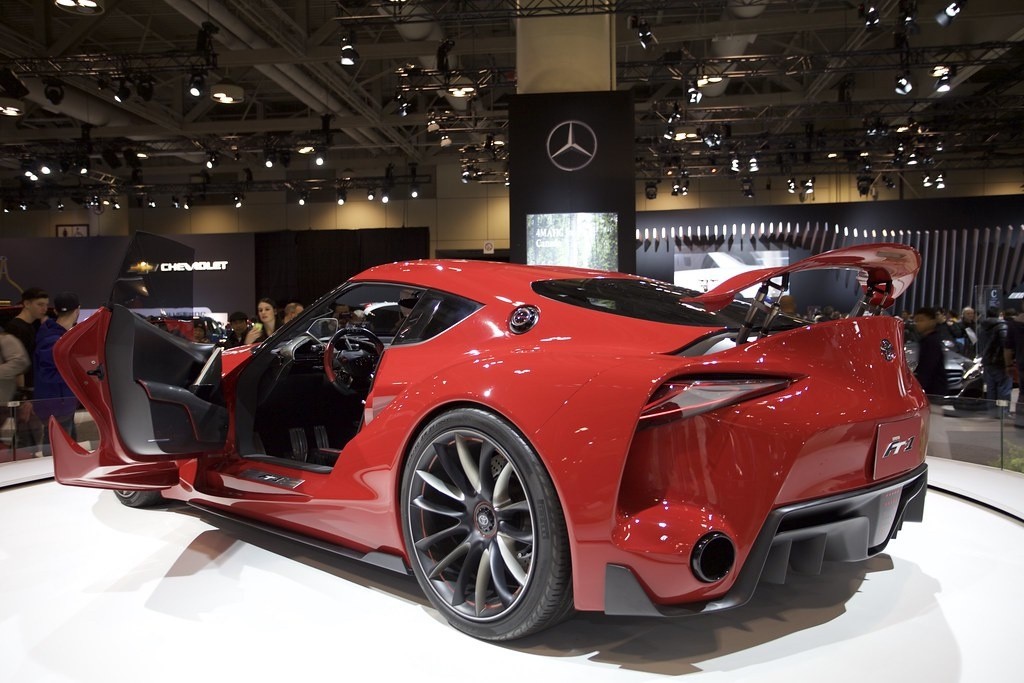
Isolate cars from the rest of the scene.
[0,300,255,345]
[901,329,986,399]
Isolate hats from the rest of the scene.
[10,287,48,305]
[53,292,80,313]
[229,312,249,322]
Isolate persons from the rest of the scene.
[778,294,1024,419]
[0,287,416,458]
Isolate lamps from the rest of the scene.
[637,0,968,200]
[0,0,510,214]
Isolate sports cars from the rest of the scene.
[47,241,933,646]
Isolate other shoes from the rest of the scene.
[1008,413,1017,419]
[1000,407,1008,414]
